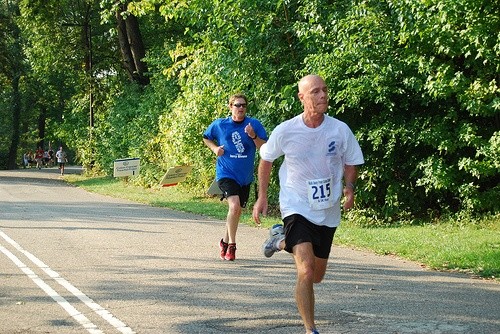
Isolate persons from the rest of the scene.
[202,95,268,261]
[252,74,365,334]
[24,146,67,175]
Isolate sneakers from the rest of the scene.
[219,238,237,261]
[263,223,285,258]
[304,329,322,334]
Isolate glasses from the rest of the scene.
[234,103,248,108]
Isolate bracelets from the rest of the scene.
[347,180,356,191]
[253,134,257,139]
